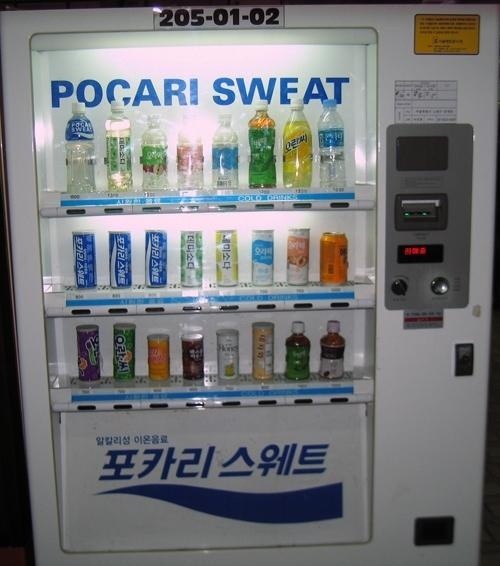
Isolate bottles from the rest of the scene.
[283,97,313,189]
[319,320,345,378]
[248,99,277,188]
[176,128,205,191]
[141,114,168,191]
[105,100,133,193]
[211,112,239,190]
[285,322,311,380]
[65,103,96,192]
[318,99,346,191]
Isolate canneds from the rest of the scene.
[320,233,348,284]
